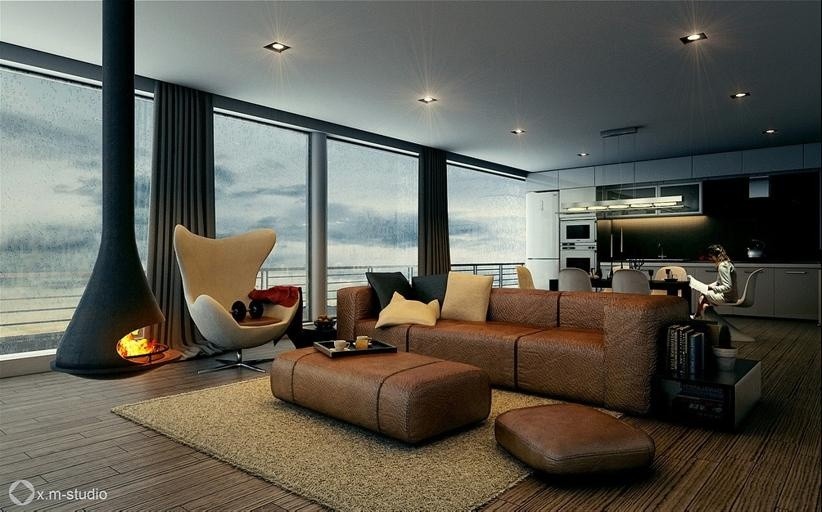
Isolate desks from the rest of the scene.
[549,278,691,315]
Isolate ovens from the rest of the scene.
[560,246,596,276]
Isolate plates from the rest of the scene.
[664,279,678,281]
[352,342,373,346]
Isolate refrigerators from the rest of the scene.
[525,190,559,292]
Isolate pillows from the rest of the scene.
[375,291,440,328]
[411,274,447,309]
[366,272,412,310]
[441,271,494,322]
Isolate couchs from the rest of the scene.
[337,285,689,417]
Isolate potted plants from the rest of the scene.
[712,324,739,371]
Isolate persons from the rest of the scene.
[688,244,739,320]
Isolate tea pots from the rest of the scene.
[746,239,767,258]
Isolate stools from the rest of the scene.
[495,403,655,488]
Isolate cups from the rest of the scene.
[355,339,368,351]
[357,336,373,343]
[333,339,350,351]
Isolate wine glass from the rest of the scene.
[649,269,654,283]
[665,269,672,279]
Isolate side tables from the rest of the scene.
[653,358,762,425]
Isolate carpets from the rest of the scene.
[111,375,624,512]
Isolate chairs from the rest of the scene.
[701,268,764,343]
[651,266,688,297]
[517,266,535,289]
[612,269,650,295]
[558,268,592,291]
[173,224,300,374]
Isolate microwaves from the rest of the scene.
[559,220,597,242]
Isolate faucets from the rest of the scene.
[658,242,664,259]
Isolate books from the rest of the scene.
[686,274,709,296]
[666,323,726,430]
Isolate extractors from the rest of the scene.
[749,176,771,198]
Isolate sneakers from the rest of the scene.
[690,314,702,320]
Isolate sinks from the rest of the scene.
[625,258,690,262]
